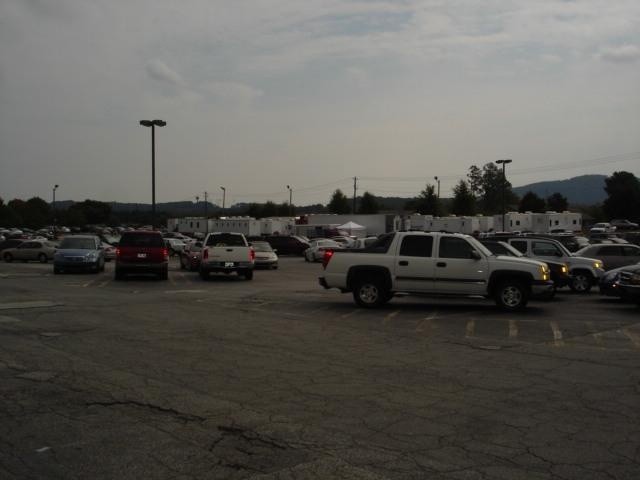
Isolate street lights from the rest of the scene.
[435,176,441,198]
[141,117,169,231]
[286,184,293,216]
[52,184,60,238]
[496,159,513,232]
[220,186,226,216]
